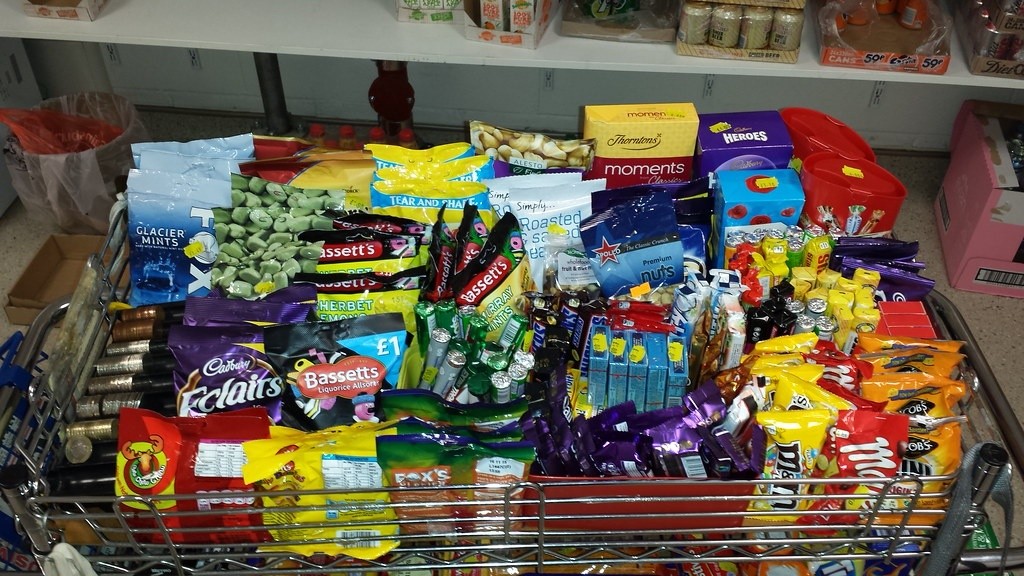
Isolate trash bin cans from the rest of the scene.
[5,92,151,236]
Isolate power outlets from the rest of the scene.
[703,73,716,98]
[870,80,888,109]
[543,70,554,91]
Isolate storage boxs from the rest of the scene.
[665,334,689,408]
[676,0,807,64]
[395,0,465,25]
[572,379,592,419]
[628,330,648,413]
[525,471,759,533]
[561,0,679,42]
[884,313,934,327]
[947,0,1024,80]
[694,112,795,177]
[588,324,612,403]
[24,0,111,21]
[929,98,1024,300]
[590,394,607,417]
[876,298,927,315]
[812,0,951,76]
[888,325,939,340]
[585,100,699,185]
[645,332,668,413]
[565,367,582,405]
[710,166,807,269]
[608,330,629,408]
[463,0,561,50]
[7,233,129,332]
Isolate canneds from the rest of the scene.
[678,1,805,50]
[958,0,1024,61]
[823,0,930,34]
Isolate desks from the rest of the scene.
[0,0,1024,136]
